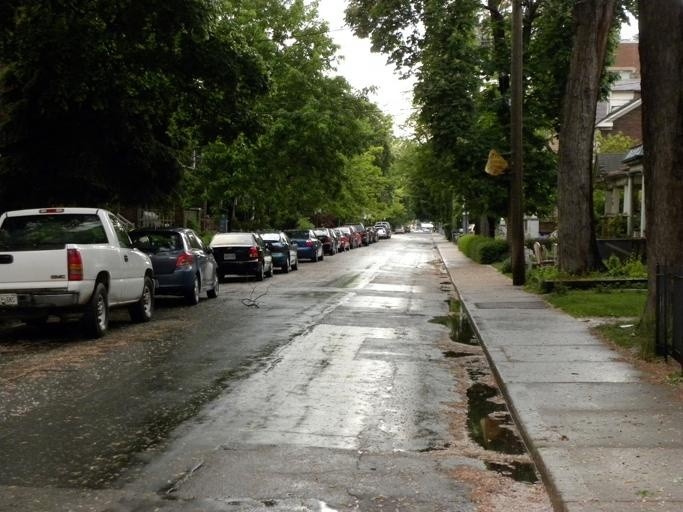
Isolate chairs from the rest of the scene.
[534,242,555,268]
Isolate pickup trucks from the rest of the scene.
[0,202,159,338]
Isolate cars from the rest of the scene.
[132,225,220,306]
[393,225,431,234]
[208,220,393,282]
